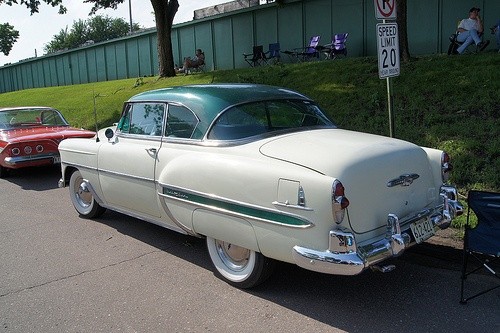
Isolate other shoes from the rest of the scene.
[479,39,490,49]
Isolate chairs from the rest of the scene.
[320,33,349,64]
[293,35,321,62]
[460,190,500,306]
[243,45,263,68]
[449,30,483,56]
[188,52,206,75]
[260,43,280,66]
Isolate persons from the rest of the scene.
[452,7,490,56]
[177,49,204,75]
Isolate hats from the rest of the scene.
[470,7,480,12]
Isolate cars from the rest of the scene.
[57,82,465,290]
[0,106,97,179]
